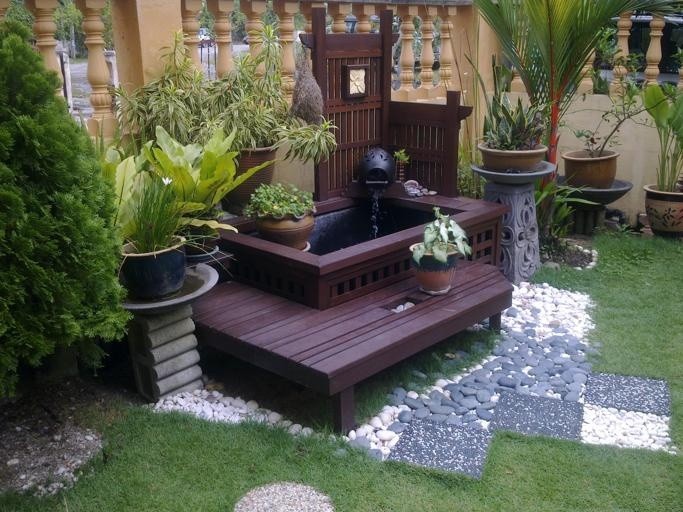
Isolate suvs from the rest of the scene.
[598,11,682,90]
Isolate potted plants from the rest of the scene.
[407,205,472,295]
[108,24,339,262]
[560,52,678,188]
[639,82,683,237]
[114,163,240,298]
[476,83,557,167]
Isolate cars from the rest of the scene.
[296,30,306,43]
[199,29,214,50]
[242,35,248,43]
